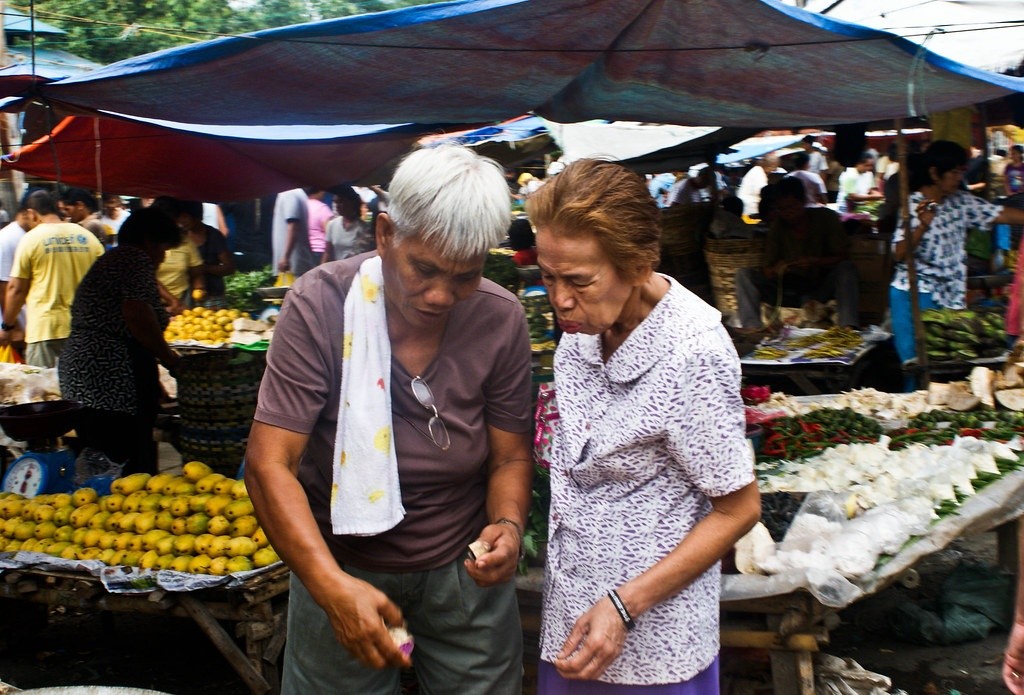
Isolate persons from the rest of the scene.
[0,182,388,369]
[503,160,548,266]
[1001,516,1024,695]
[667,126,1024,390]
[60,209,183,475]
[243,143,537,695]
[529,158,761,695]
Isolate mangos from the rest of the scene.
[192,289,203,298]
[0,461,284,577]
[163,305,251,345]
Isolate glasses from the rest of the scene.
[391,374,451,453]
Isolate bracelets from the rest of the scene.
[1,322,15,331]
[499,518,525,561]
[609,590,635,629]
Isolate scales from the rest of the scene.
[0,400,114,500]
[514,265,540,288]
[968,276,1008,307]
[253,286,290,317]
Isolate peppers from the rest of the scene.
[753,407,1023,451]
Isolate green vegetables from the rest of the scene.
[516,466,552,577]
[224,262,274,307]
[483,251,519,290]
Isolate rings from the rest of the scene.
[1012,672,1018,678]
